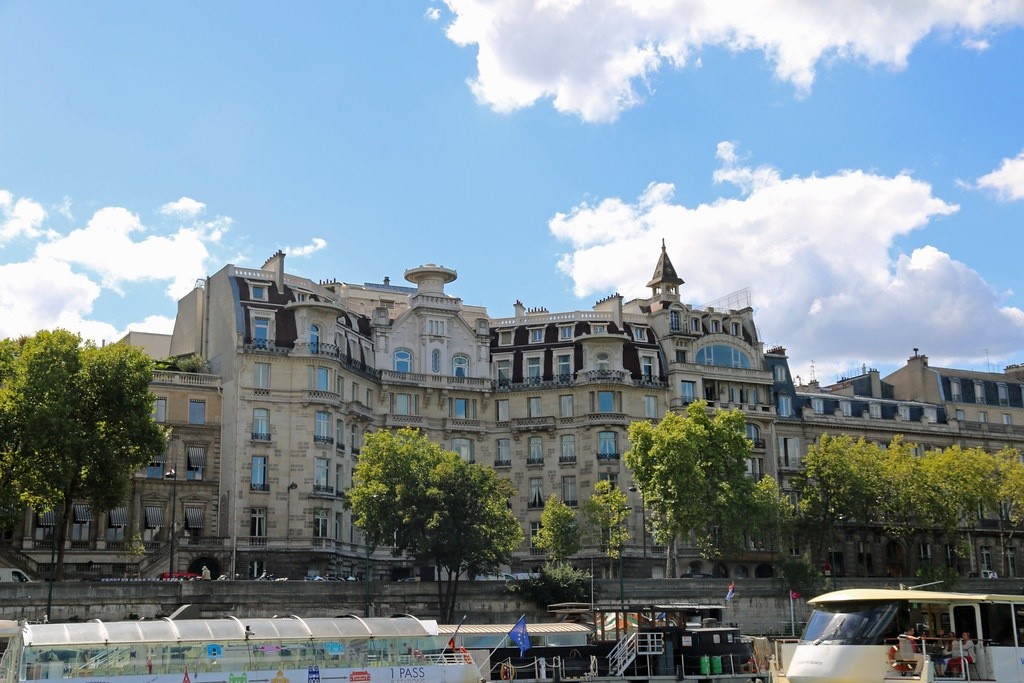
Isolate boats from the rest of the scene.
[2,588,1024,682]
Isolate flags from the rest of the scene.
[725,582,735,601]
[448,616,466,651]
[791,591,801,599]
[507,614,532,659]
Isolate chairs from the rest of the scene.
[965,646,982,681]
[896,660,919,676]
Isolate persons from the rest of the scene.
[257,569,269,582]
[932,631,960,677]
[988,569,998,579]
[355,571,362,581]
[914,623,949,663]
[196,565,211,581]
[948,632,977,678]
[394,573,422,582]
[895,568,903,578]
[12,572,20,582]
[898,624,925,677]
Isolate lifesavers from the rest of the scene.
[500,664,515,680]
[889,642,919,672]
[460,646,472,663]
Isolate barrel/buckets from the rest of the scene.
[703,618,717,628]
[700,654,740,674]
